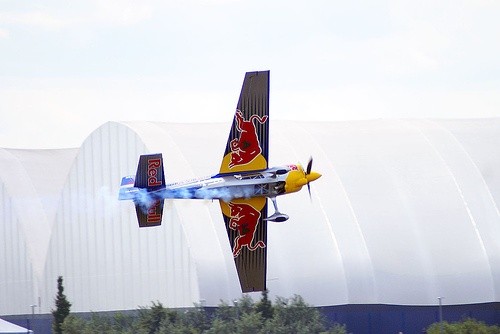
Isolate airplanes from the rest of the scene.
[118,70,323,293]
